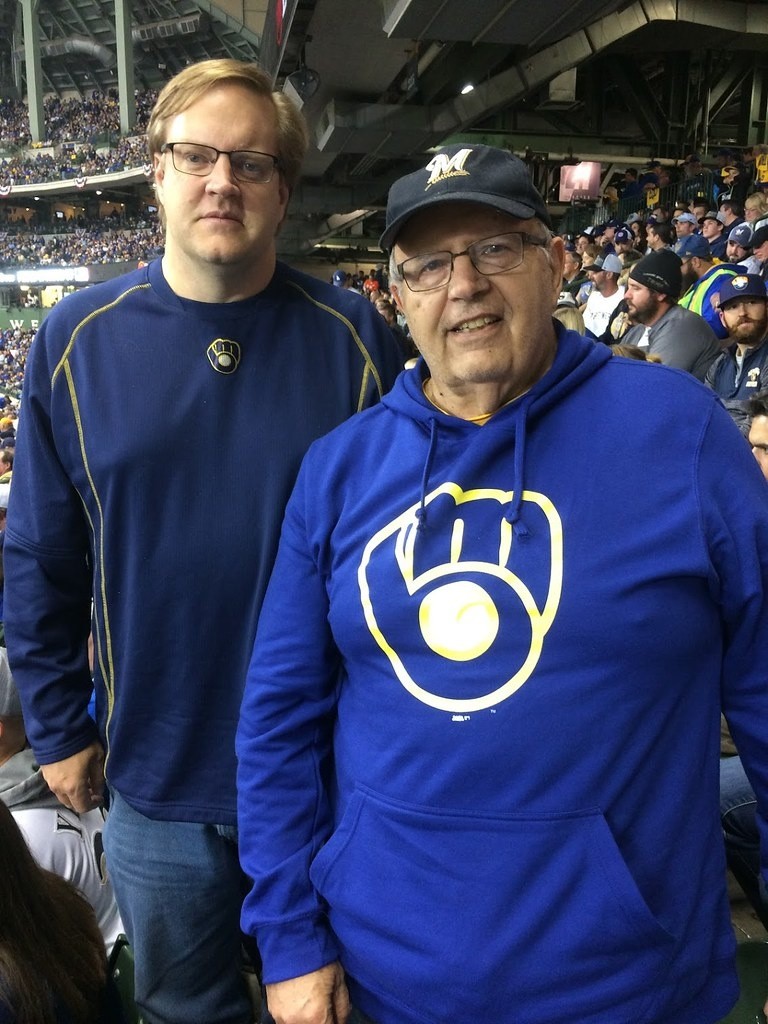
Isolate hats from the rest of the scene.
[713,148,731,157]
[671,212,698,225]
[0,431,14,439]
[603,219,619,227]
[21,356,25,361]
[584,253,623,273]
[725,161,746,174]
[614,223,629,234]
[680,155,700,167]
[333,271,347,287]
[15,372,24,381]
[623,212,641,224]
[584,226,605,236]
[0,437,15,449]
[379,144,554,249]
[698,211,726,225]
[751,216,768,247]
[677,234,710,257]
[556,291,579,309]
[718,274,768,306]
[646,213,657,224]
[0,646,23,717]
[646,161,661,171]
[0,398,6,408]
[614,228,635,241]
[725,223,754,248]
[630,250,683,297]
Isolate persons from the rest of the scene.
[24,291,41,308]
[550,145,768,487]
[2,58,420,1024]
[704,273,768,439]
[0,205,166,270]
[619,248,724,384]
[0,648,125,1024]
[0,327,39,558]
[328,264,418,356]
[234,142,768,1024]
[0,86,159,187]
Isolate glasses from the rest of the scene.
[397,231,547,292]
[680,258,692,267]
[744,207,759,213]
[160,142,284,185]
